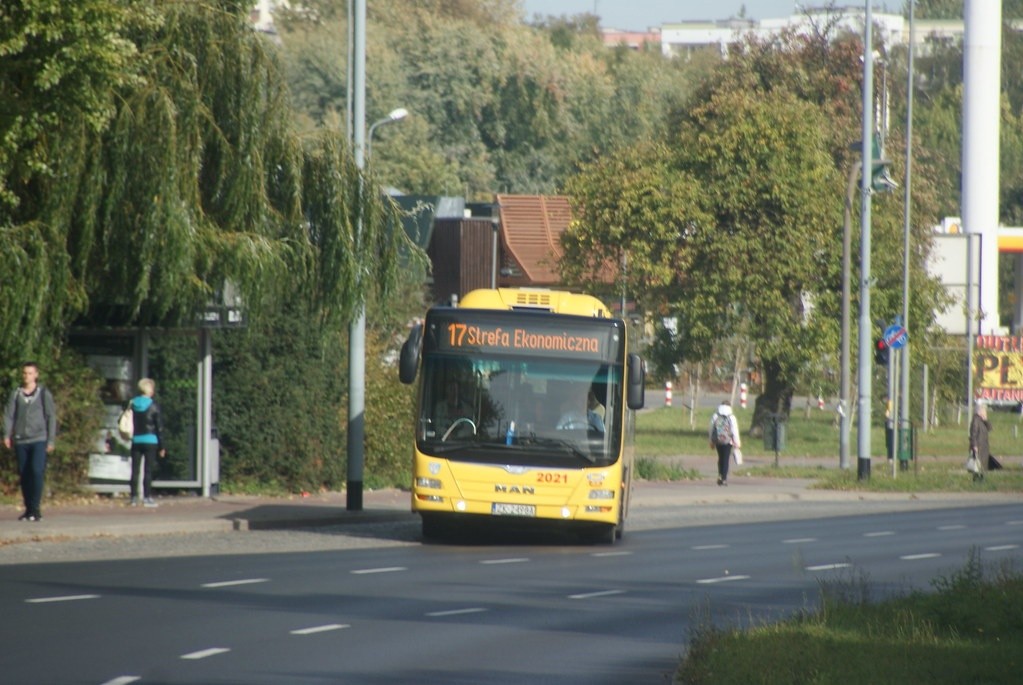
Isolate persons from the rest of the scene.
[968,408,992,481]
[117,378,167,507]
[884,400,894,464]
[434,380,476,440]
[555,391,606,432]
[106,432,130,456]
[708,401,741,487]
[2,362,57,522]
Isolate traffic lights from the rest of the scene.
[874,336,890,365]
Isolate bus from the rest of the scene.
[400,286,648,545]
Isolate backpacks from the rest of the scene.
[712,414,733,446]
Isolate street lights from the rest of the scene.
[837,157,894,468]
[346,108,410,511]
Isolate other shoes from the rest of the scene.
[718,478,728,486]
[18,513,43,521]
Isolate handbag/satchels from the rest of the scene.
[733,445,743,465]
[966,448,981,473]
[118,400,134,440]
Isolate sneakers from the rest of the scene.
[130,496,137,506]
[142,497,159,508]
